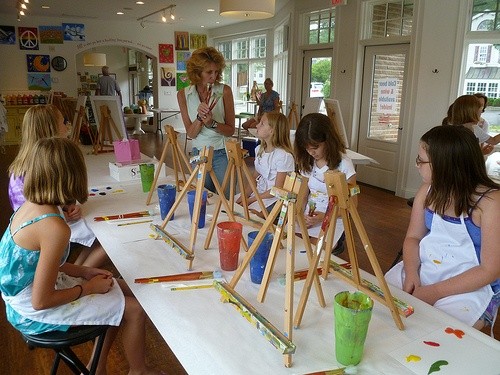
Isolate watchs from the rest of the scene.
[204,119,218,129]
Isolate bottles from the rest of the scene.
[0,92,47,105]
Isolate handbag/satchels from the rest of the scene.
[112,138,140,162]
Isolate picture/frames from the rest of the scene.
[98,73,116,81]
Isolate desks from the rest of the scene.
[246,101,261,114]
[124,113,153,135]
[76,142,500,375]
[150,108,182,136]
[235,114,254,139]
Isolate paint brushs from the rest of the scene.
[134,270,223,284]
[200,85,223,126]
[93,209,156,221]
[276,262,352,284]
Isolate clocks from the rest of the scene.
[52,56,68,71]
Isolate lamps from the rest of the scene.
[161,5,176,22]
[219,0,275,19]
[139,18,145,30]
[84,48,107,67]
[16,0,30,22]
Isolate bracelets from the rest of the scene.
[197,115,202,121]
[74,285,82,300]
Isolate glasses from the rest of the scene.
[417,154,431,167]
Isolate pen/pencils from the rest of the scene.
[117,220,153,226]
[171,284,215,291]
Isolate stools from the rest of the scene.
[22,322,105,375]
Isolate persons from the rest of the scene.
[177,47,236,201]
[96,65,123,107]
[473,93,490,134]
[242,78,279,137]
[230,111,296,225]
[442,95,500,161]
[294,113,358,253]
[10,104,107,268]
[384,125,500,331]
[0,137,162,375]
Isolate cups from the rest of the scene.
[188,190,207,228]
[217,221,243,271]
[334,291,374,366]
[140,164,155,193]
[248,230,274,283]
[157,185,176,221]
